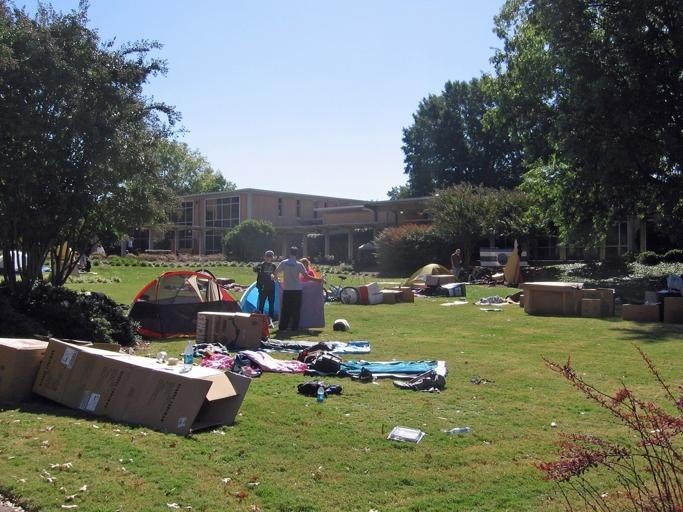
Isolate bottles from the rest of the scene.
[317,385,324,402]
[449,427,471,434]
[184,341,193,369]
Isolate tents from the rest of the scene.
[238,280,279,322]
[403,263,451,287]
[126,269,242,340]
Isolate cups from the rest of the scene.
[167,358,178,365]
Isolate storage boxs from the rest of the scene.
[360,274,466,307]
[32,338,253,437]
[0,336,124,405]
[522,281,683,326]
[196,312,267,348]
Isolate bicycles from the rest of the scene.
[322,268,360,305]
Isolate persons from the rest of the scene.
[80,257,91,272]
[253,250,278,318]
[273,247,326,331]
[97,244,105,254]
[450,249,463,275]
